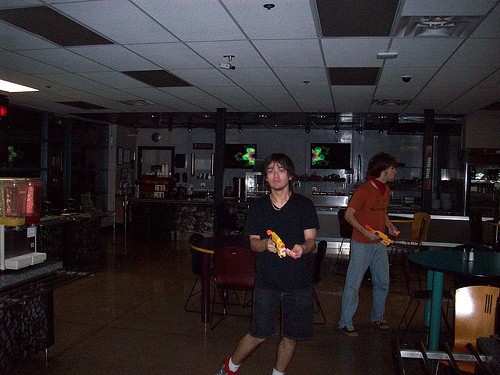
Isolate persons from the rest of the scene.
[338,154,400,336]
[214,153,320,375]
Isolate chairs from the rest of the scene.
[186,213,500,371]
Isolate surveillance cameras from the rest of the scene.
[220,63,235,70]
[402,76,411,82]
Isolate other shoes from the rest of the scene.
[214,353,241,375]
[371,320,390,329]
[338,326,359,336]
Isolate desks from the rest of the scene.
[410,248,500,352]
[191,236,250,322]
[389,216,414,223]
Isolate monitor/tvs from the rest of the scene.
[310,143,351,169]
[224,144,257,169]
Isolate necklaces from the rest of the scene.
[272,203,281,209]
